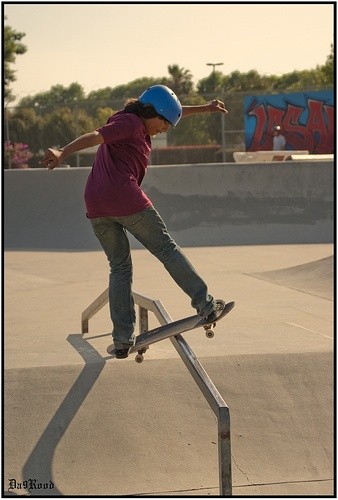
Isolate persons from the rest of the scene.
[43,84,228,360]
[272,124,286,151]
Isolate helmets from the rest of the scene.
[138,85,182,127]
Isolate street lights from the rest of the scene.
[206,62,224,94]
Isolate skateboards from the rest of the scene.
[106,301,234,361]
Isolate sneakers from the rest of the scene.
[114,333,136,359]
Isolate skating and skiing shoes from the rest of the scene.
[203,300,225,323]
